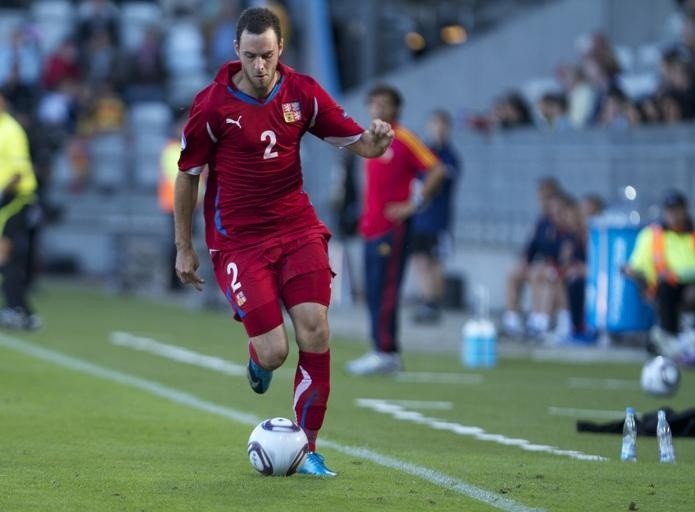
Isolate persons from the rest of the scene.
[174,8,395,476]
[629,189,695,337]
[501,175,605,349]
[341,87,461,378]
[467,30,695,130]
[0,0,243,335]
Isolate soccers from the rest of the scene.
[248,417,308,478]
[640,358,680,394]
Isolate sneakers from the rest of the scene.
[1,305,41,332]
[649,323,695,364]
[345,350,407,375]
[496,312,552,343]
[246,353,274,395]
[297,451,337,480]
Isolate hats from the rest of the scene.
[659,187,686,207]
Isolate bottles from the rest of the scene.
[621,408,637,460]
[656,409,676,464]
